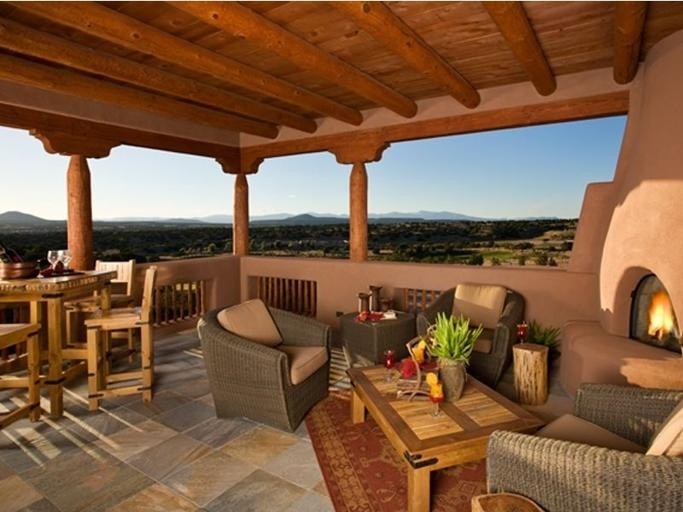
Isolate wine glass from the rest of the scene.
[46,250,62,274]
[516,321,529,347]
[382,349,396,384]
[62,249,74,276]
[427,382,446,418]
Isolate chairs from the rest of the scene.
[83,265,158,410]
[62,259,134,372]
[0,321,41,433]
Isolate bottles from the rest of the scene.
[1,244,23,264]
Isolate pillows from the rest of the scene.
[450,283,506,331]
[216,300,283,349]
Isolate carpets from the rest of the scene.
[301,388,522,512]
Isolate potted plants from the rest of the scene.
[419,312,484,402]
[526,320,561,388]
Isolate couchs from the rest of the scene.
[416,284,525,388]
[198,298,332,431]
[485,383,682,512]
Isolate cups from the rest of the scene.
[357,285,382,315]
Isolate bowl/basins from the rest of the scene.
[0,260,40,270]
[0,268,40,280]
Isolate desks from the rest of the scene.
[340,310,415,369]
